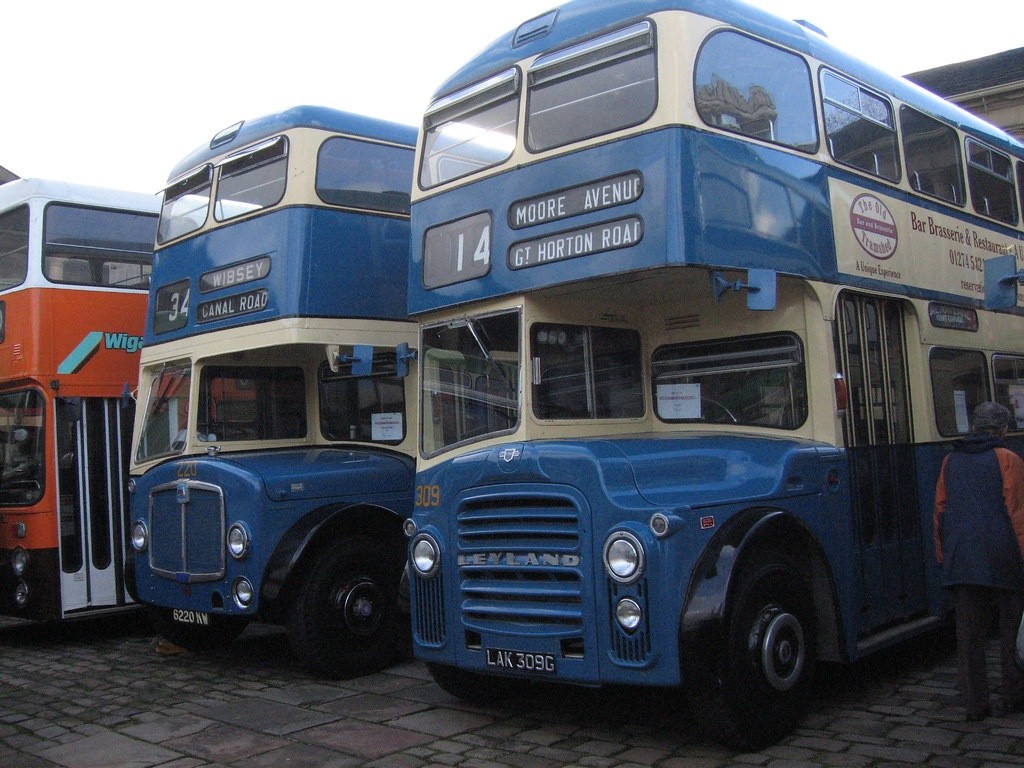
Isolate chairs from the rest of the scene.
[738,118,993,217]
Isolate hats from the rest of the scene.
[973,402,1011,429]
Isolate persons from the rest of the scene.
[935,402,1024,723]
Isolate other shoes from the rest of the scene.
[1002,688,1024,714]
[965,707,992,723]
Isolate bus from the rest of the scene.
[400,1,1024,754]
[122,104,598,681]
[0,177,294,633]
